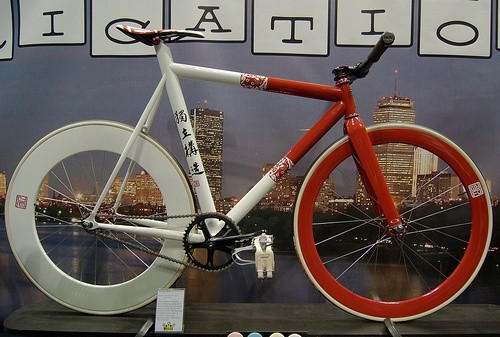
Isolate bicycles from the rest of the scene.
[4,25,494,322]
